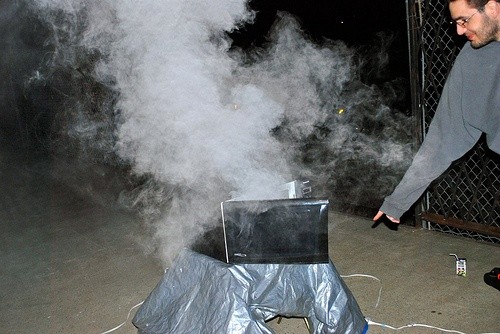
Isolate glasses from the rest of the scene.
[450,8,481,27]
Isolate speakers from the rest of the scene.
[190,199,329,265]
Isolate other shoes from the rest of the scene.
[484,272,500,291]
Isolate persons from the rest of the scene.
[373,0,500,224]
